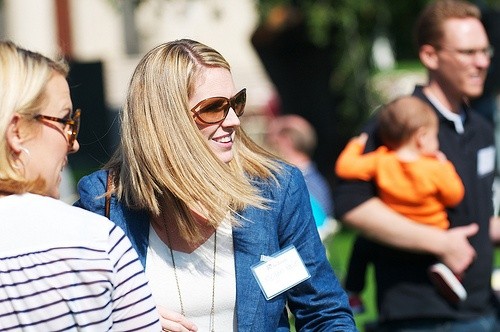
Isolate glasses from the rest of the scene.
[31,108,81,149]
[190,88,248,125]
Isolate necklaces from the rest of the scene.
[160,202,217,332]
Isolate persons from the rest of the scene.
[333,96,467,314]
[77,38,357,332]
[0,0,331,237]
[0,39,162,332]
[333,0,500,332]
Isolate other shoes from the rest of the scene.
[346,293,366,315]
[431,263,467,310]
